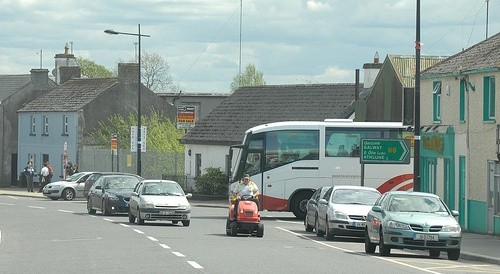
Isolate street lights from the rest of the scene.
[104,23,151,176]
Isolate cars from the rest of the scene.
[129,180,192,226]
[42,172,101,201]
[365,191,462,261]
[83,173,146,216]
[305,185,383,241]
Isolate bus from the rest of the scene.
[229,119,414,219]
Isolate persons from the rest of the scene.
[245,143,361,176]
[231,173,261,220]
[38,163,49,192]
[23,161,37,192]
[44,161,54,183]
[232,177,260,219]
[66,161,79,179]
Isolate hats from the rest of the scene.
[241,174,250,180]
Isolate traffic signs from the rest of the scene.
[360,138,410,164]
